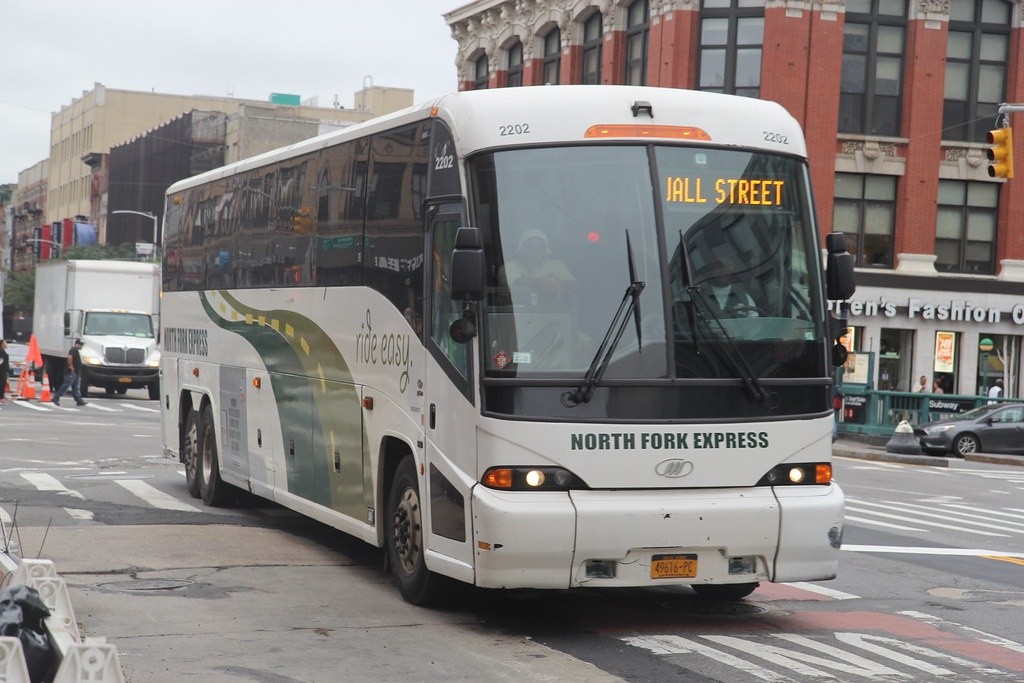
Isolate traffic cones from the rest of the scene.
[16,368,25,394]
[27,372,38,399]
[23,372,29,397]
[37,373,52,402]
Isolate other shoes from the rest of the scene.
[0,398,9,405]
[77,401,88,406]
[51,398,60,405]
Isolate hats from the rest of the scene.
[75,338,85,344]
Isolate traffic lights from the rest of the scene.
[987,126,1015,179]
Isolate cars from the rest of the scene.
[5,343,32,377]
[913,401,1024,458]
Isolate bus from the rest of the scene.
[160,84,854,607]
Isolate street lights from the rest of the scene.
[979,338,994,405]
[111,210,158,262]
[26,238,64,261]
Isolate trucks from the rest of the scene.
[33,261,163,400]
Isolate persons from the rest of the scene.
[0,339,9,403]
[497,228,580,307]
[986,379,1004,405]
[51,338,88,407]
[932,380,945,394]
[695,255,762,318]
[913,375,930,394]
